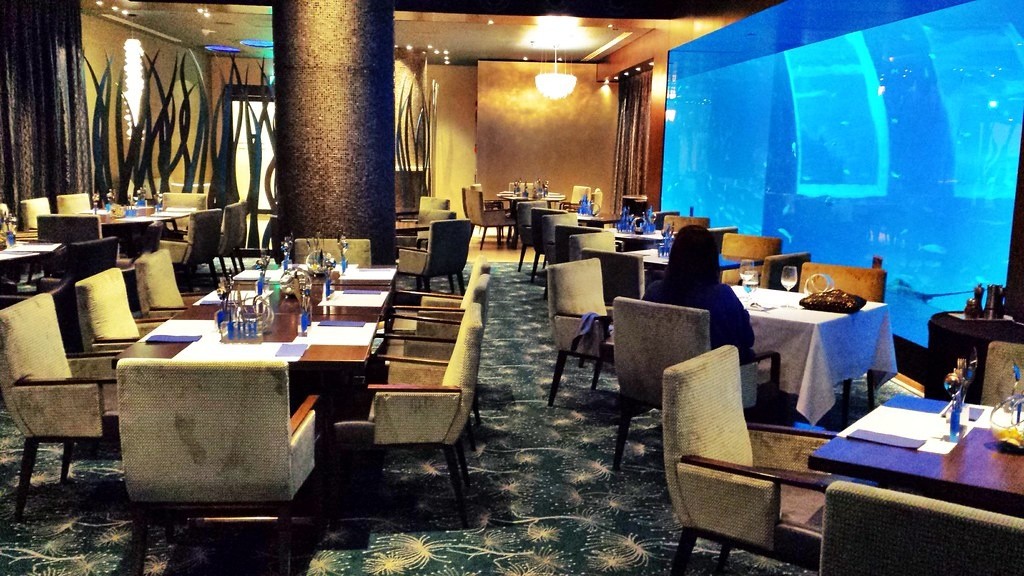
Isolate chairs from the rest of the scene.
[0,181,1024,576]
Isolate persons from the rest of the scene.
[641,225,755,358]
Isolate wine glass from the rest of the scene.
[779,266,798,308]
[743,271,759,303]
[739,259,754,295]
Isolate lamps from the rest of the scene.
[124,13,145,93]
[534,44,577,99]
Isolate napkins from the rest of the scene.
[946,399,1021,430]
[295,321,377,347]
[281,263,358,275]
[80,210,108,215]
[170,341,307,363]
[151,212,189,217]
[166,207,197,212]
[139,319,222,341]
[116,216,169,221]
[232,269,282,281]
[12,243,62,251]
[120,205,153,209]
[318,290,389,307]
[192,289,273,305]
[837,407,970,457]
[338,268,396,281]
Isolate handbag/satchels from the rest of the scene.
[800,287,867,314]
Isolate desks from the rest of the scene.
[724,284,900,426]
[623,245,761,280]
[805,394,1024,509]
[114,261,399,423]
[573,216,622,229]
[497,190,567,250]
[84,206,198,255]
[0,241,66,303]
[612,226,675,251]
